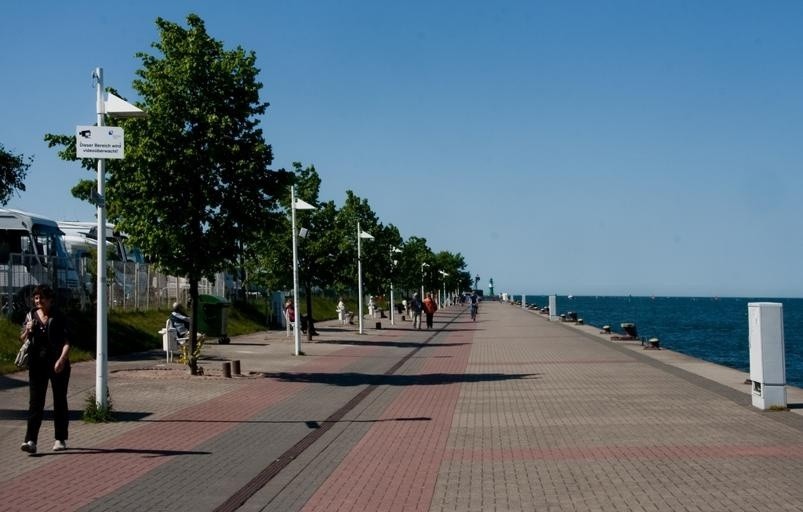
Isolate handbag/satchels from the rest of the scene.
[14,311,33,368]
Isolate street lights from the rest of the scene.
[421,272,427,313]
[293,226,308,357]
[390,260,399,325]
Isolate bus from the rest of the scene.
[0,207,149,301]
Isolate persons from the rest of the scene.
[19,283,72,454]
[373,302,388,318]
[410,291,426,331]
[284,297,321,338]
[423,291,437,331]
[468,291,479,320]
[337,297,355,325]
[168,301,206,359]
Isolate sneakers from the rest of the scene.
[412,323,433,329]
[52,441,66,451]
[312,332,320,336]
[21,441,37,454]
